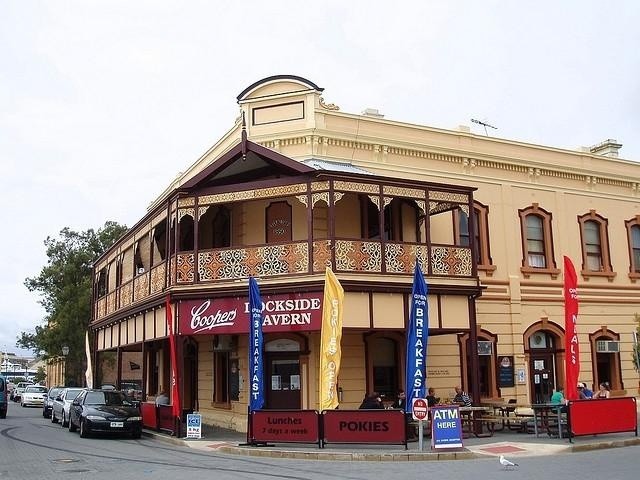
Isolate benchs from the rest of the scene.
[408,397,572,438]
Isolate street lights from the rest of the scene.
[4,354,10,380]
[25,362,30,382]
[62,345,70,386]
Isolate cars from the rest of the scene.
[68,389,144,439]
[99,382,117,391]
[41,385,71,418]
[20,385,49,407]
[51,387,86,427]
[10,387,15,400]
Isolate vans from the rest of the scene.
[0,374,8,419]
[120,382,143,396]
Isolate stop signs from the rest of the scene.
[412,398,428,421]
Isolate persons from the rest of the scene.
[425,387,440,420]
[125,390,136,404]
[156,388,169,408]
[551,384,568,414]
[452,386,472,415]
[576,383,591,400]
[581,383,592,399]
[291,385,295,390]
[594,383,610,399]
[359,390,407,410]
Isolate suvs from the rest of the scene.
[12,382,34,401]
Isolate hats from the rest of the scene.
[577,383,584,388]
